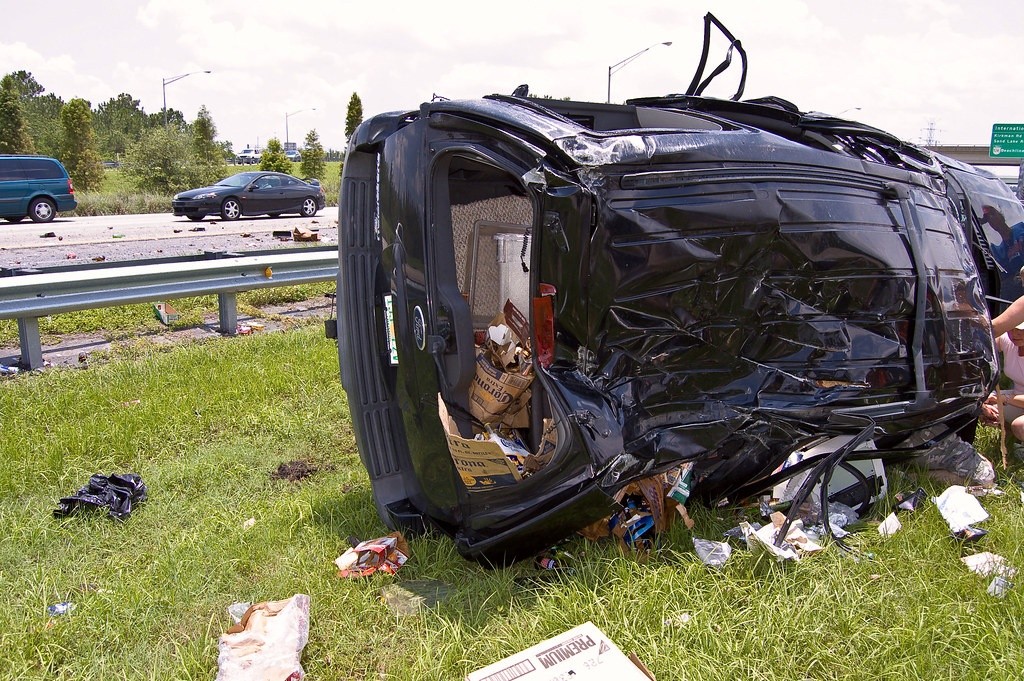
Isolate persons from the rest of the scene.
[977,205,1024,267]
[261,177,271,188]
[988,267,1024,455]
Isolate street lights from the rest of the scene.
[163,71,211,133]
[286,108,316,150]
[608,42,672,104]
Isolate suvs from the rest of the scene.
[0,154,77,223]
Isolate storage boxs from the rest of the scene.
[466,220,531,327]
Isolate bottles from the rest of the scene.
[534,555,573,574]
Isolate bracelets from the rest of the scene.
[1002,393,1010,405]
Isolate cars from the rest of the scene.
[102,159,123,168]
[235,148,264,164]
[285,151,302,162]
[326,13,1024,570]
[171,171,325,221]
